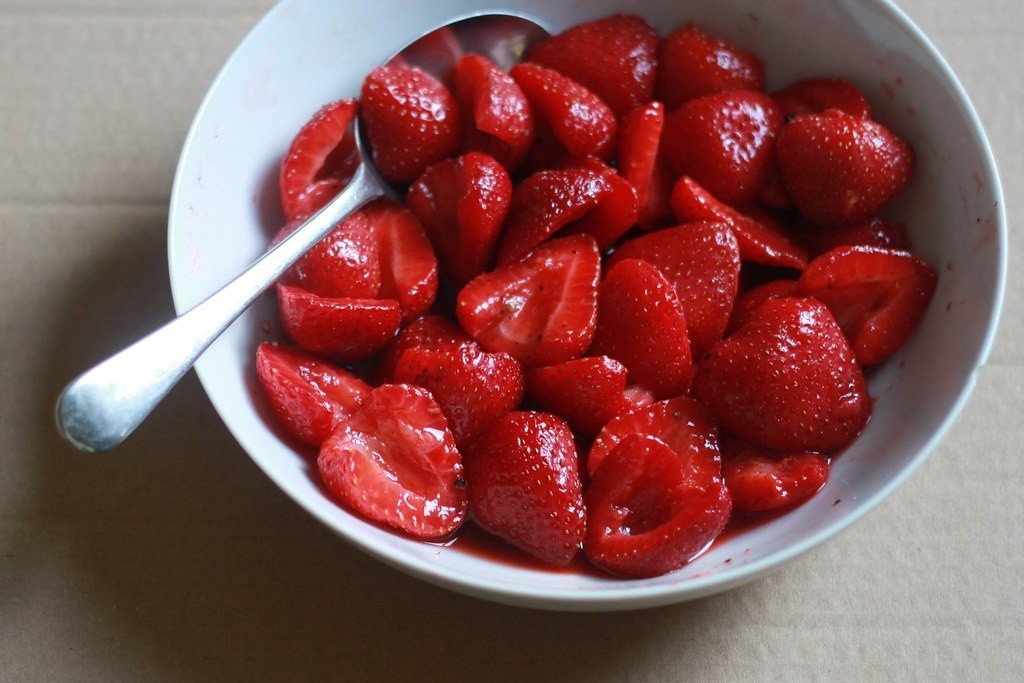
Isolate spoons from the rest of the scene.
[57,13,553,454]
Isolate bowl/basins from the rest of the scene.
[168,0,1007,613]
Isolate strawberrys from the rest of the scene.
[258,14,935,579]
